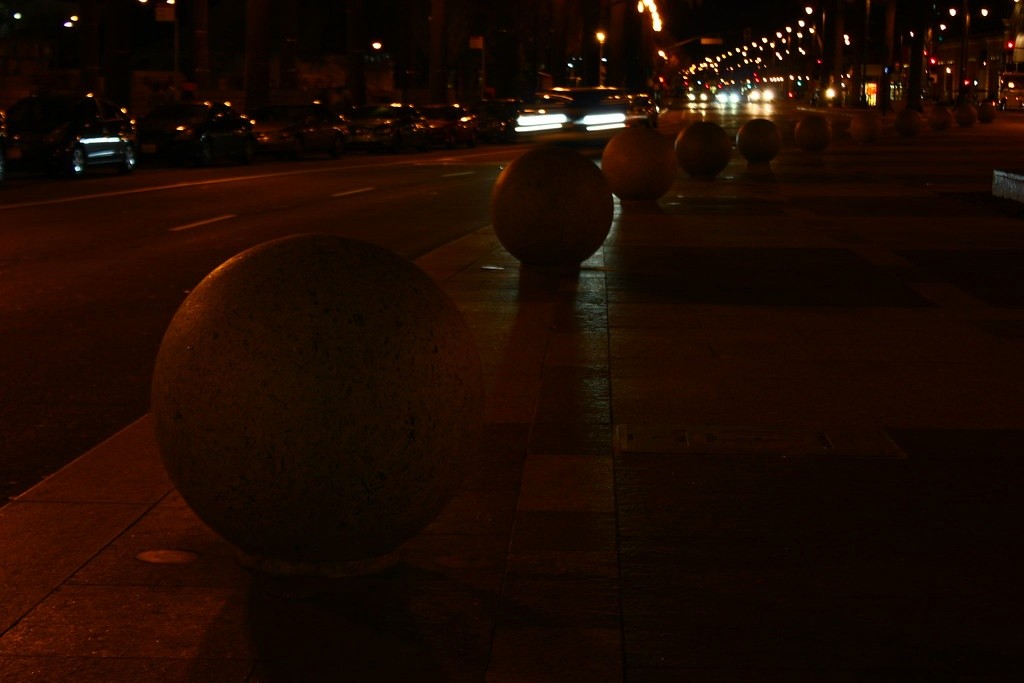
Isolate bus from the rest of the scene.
[998,70,1024,111]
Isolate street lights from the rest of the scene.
[371,42,382,108]
[596,32,605,85]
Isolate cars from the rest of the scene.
[177,85,651,166]
[0,88,260,178]
[686,87,777,102]
